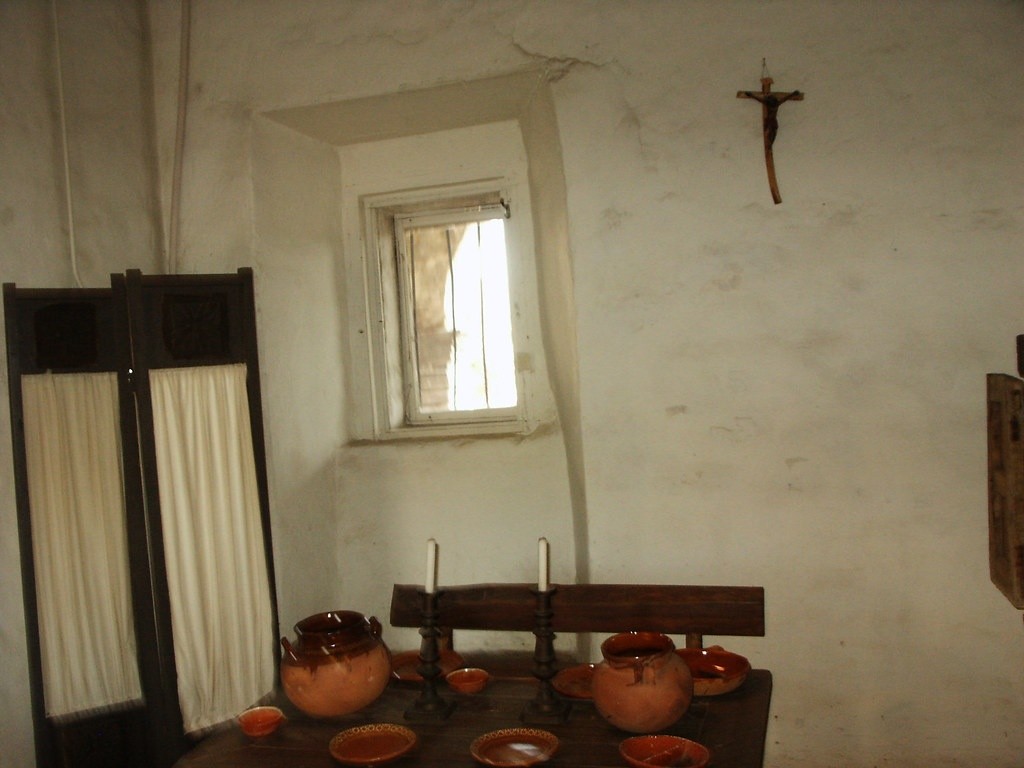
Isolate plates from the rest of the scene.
[329,724,417,766]
[469,728,559,766]
[549,662,596,699]
[391,650,463,681]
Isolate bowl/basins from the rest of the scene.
[237,706,283,736]
[674,648,751,696]
[620,735,709,768]
[445,667,490,695]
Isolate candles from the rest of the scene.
[424,538,436,594]
[537,537,548,591]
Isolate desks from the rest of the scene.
[176,651,772,768]
